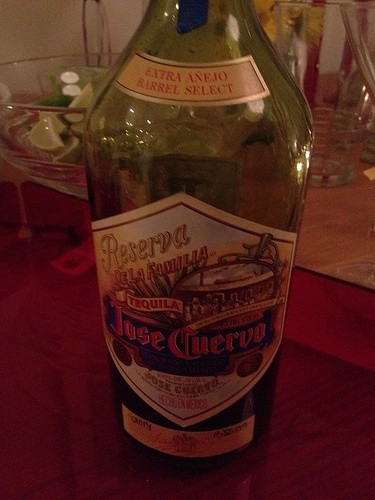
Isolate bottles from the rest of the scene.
[80,0,315,476]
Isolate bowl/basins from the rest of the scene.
[0,54,125,201]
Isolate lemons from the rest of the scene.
[29,83,93,164]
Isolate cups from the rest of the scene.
[269,2,373,187]
